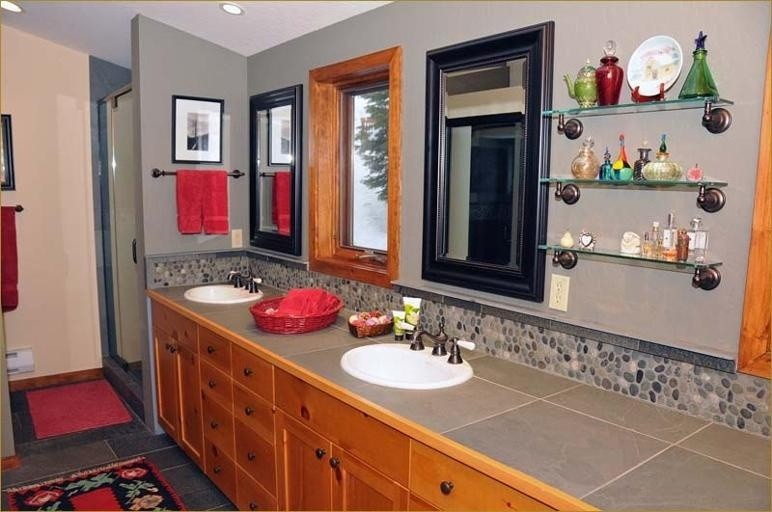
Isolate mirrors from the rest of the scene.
[248,83,305,257]
[416,18,555,304]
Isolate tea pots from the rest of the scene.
[562,55,598,108]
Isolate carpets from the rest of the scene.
[24,380,135,441]
[199,325,279,511]
[7,454,190,511]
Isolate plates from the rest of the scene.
[625,35,684,96]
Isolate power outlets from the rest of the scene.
[547,273,572,314]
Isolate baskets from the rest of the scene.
[250,297,343,334]
[347,319,393,337]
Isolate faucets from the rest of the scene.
[409,323,447,357]
[226,270,256,293]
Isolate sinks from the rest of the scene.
[339,343,474,391]
[183,284,265,305]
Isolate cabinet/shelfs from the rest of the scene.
[151,298,205,474]
[409,437,558,511]
[533,93,734,292]
[274,368,410,511]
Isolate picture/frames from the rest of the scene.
[0,113,18,192]
[170,94,225,166]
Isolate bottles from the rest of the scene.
[642,208,708,261]
[568,135,702,184]
[595,40,624,108]
[678,30,719,99]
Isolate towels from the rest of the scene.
[1,206,18,311]
[280,288,340,321]
[271,171,291,238]
[175,169,231,235]
